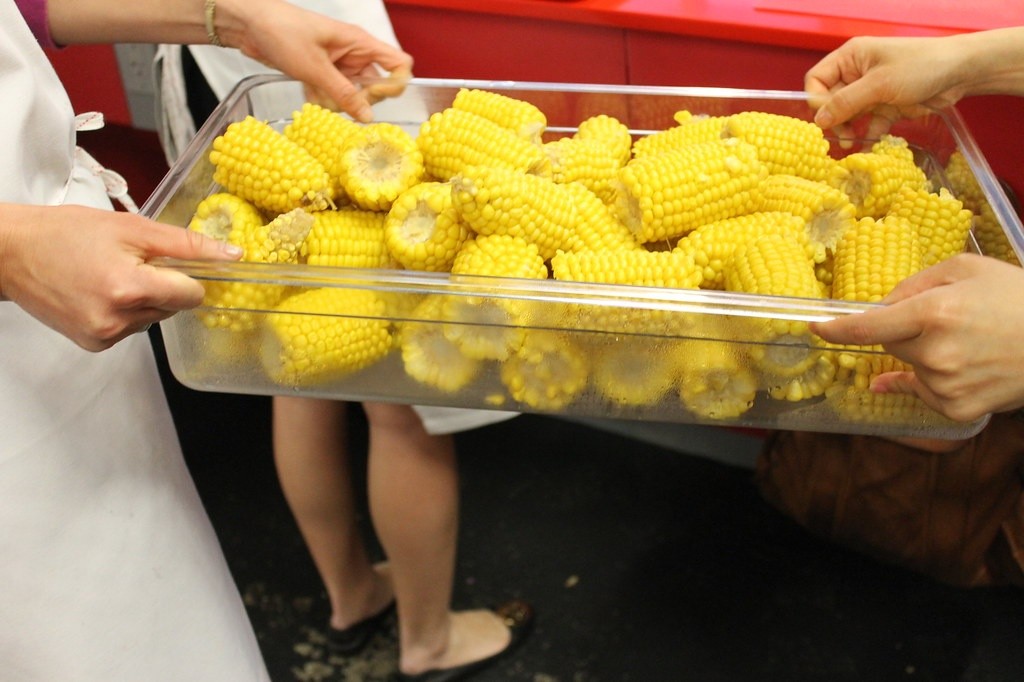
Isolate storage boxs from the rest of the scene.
[133,71,1023,440]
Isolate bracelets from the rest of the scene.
[203,0,226,48]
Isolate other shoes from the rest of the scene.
[394,600,533,682]
[326,590,397,657]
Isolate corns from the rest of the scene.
[181,87,995,428]
[946,142,1019,265]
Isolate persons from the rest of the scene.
[153,0,532,681]
[805,25,1023,421]
[0,0,413,682]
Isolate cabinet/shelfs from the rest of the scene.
[384,0,1024,471]
